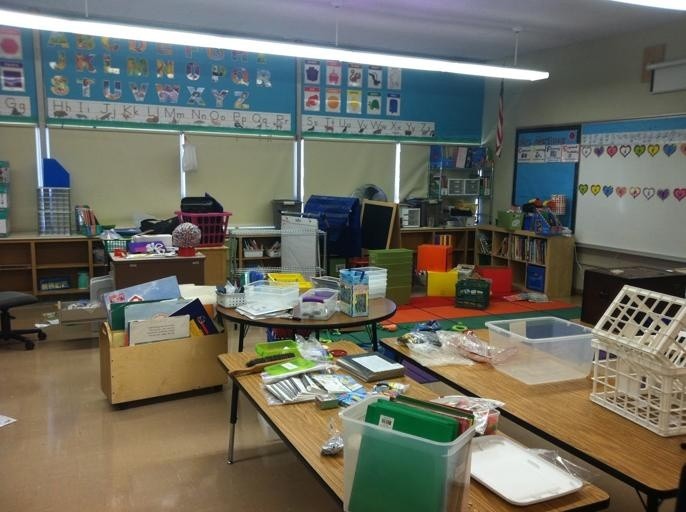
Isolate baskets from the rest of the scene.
[175,212,232,246]
[41,275,72,290]
[215,291,245,308]
[103,239,126,253]
[590,284,686,437]
[455,279,491,309]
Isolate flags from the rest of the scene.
[494,80,505,161]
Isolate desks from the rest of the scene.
[216,340,610,511]
[378,327,683,511]
[217,299,398,465]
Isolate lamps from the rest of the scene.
[0,1,551,84]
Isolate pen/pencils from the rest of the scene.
[81,210,96,228]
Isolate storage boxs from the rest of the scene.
[591,284,683,438]
[334,394,481,510]
[485,316,593,387]
[243,266,388,320]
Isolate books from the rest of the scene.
[75,206,97,234]
[347,393,475,512]
[259,350,406,407]
[429,144,491,200]
[503,292,552,303]
[236,302,295,322]
[102,275,225,348]
[434,232,546,266]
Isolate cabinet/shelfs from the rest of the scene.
[0,235,327,305]
[399,228,575,297]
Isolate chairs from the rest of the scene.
[0,291,46,349]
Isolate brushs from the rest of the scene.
[227,352,297,377]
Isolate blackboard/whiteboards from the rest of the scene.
[359,198,397,250]
[510,112,686,262]
[2,25,484,146]
